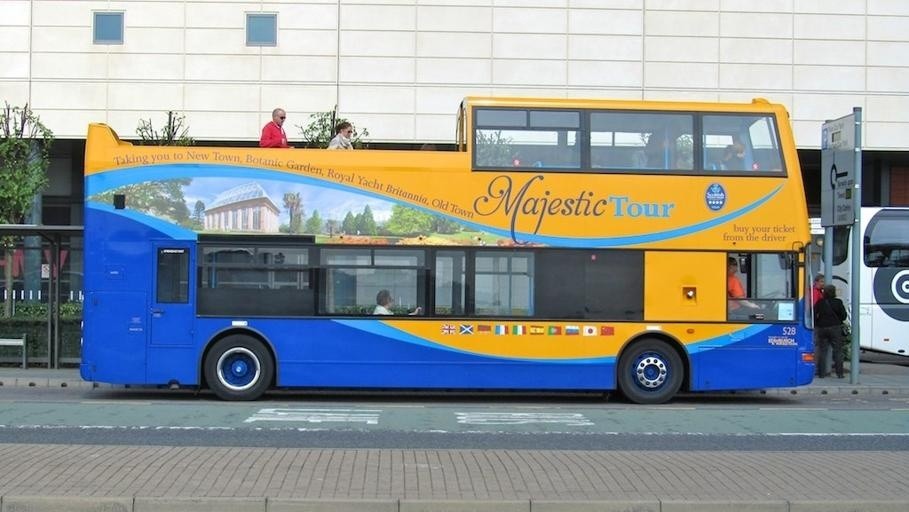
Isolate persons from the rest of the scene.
[372,289,422,316]
[814,284,848,379]
[675,142,746,170]
[805,275,825,373]
[326,122,353,149]
[259,107,295,148]
[727,257,761,316]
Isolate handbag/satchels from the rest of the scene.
[841,325,848,336]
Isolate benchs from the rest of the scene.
[0,332,28,369]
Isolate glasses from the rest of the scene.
[280,117,285,119]
[348,131,352,133]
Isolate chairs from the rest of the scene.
[708,159,727,170]
